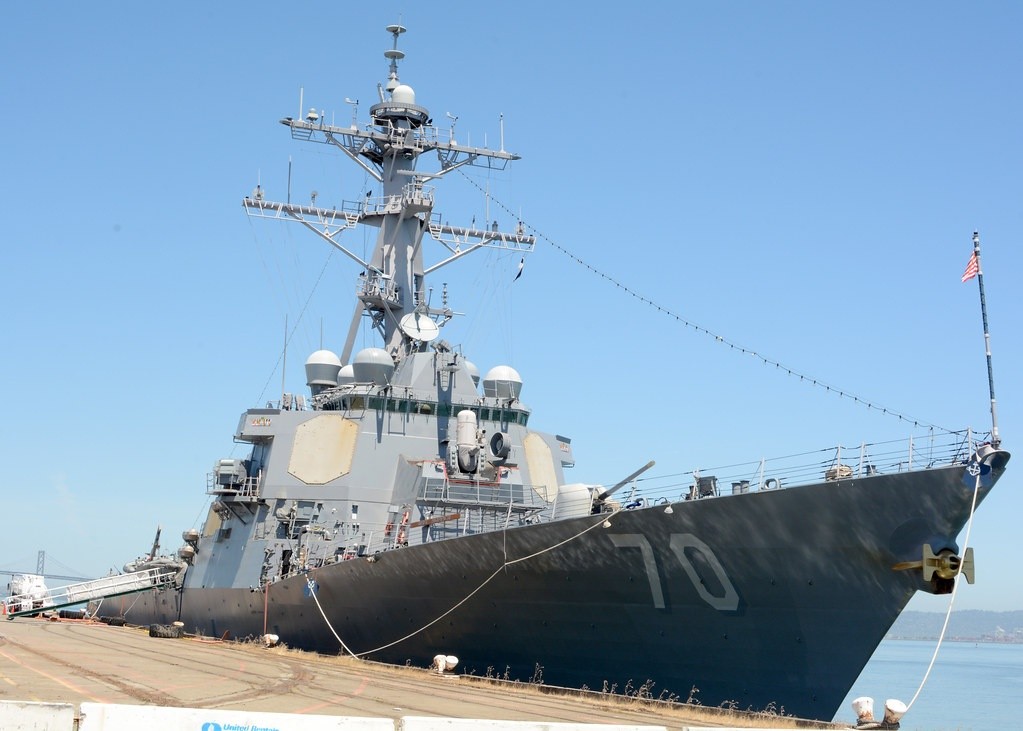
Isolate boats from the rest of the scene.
[87,26,1011,724]
[123,557,175,573]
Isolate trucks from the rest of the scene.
[2,573,54,618]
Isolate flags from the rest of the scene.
[961,250,979,282]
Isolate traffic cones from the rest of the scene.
[2,604,7,615]
[10,605,15,613]
